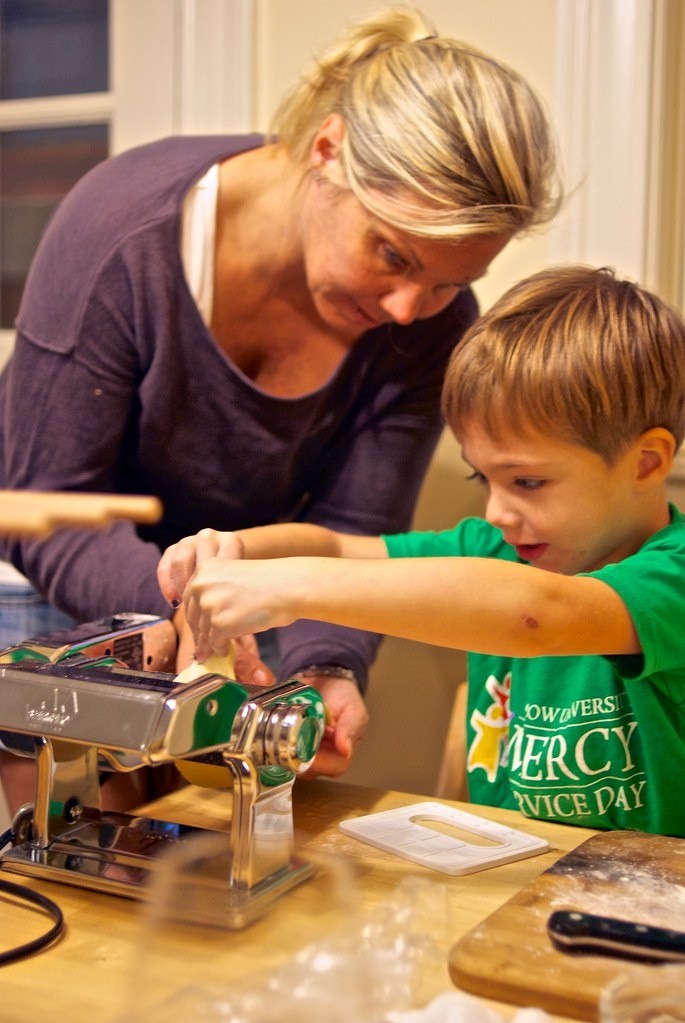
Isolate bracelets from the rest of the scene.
[287,663,360,691]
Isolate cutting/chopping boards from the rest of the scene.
[446,830,685,1023]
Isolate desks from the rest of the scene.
[0,778,601,1023]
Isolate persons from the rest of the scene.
[159,263,685,840]
[0,6,568,781]
